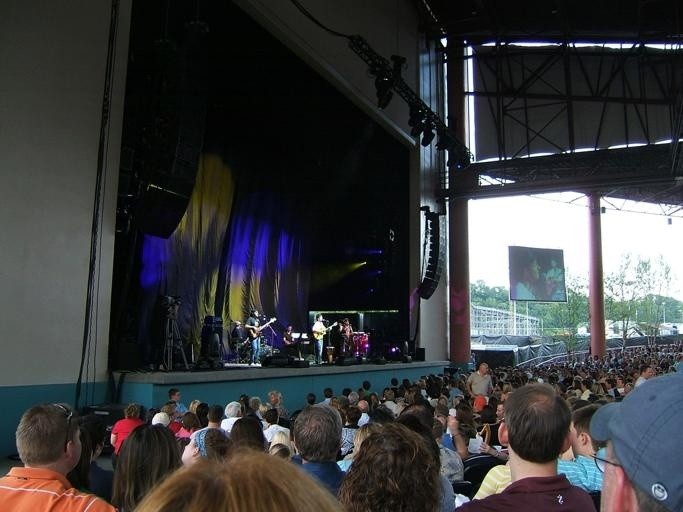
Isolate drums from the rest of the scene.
[352,331,369,357]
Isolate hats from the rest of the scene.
[589,373,683,512]
[152,412,172,427]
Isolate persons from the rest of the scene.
[312,314,332,364]
[340,318,353,353]
[546,257,565,301]
[232,322,241,337]
[511,252,541,300]
[0,341,683,512]
[283,325,304,361]
[244,310,262,367]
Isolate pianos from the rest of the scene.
[285,340,310,345]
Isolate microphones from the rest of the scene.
[323,319,328,322]
[259,314,265,317]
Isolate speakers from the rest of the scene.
[261,356,294,367]
[84,402,143,454]
[112,342,145,370]
[336,357,353,366]
[376,358,386,365]
[416,348,425,361]
[444,367,458,379]
[418,211,446,299]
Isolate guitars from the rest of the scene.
[249,317,276,339]
[313,322,339,340]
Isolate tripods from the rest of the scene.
[163,318,189,371]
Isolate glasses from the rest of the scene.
[589,447,623,473]
[51,403,73,454]
